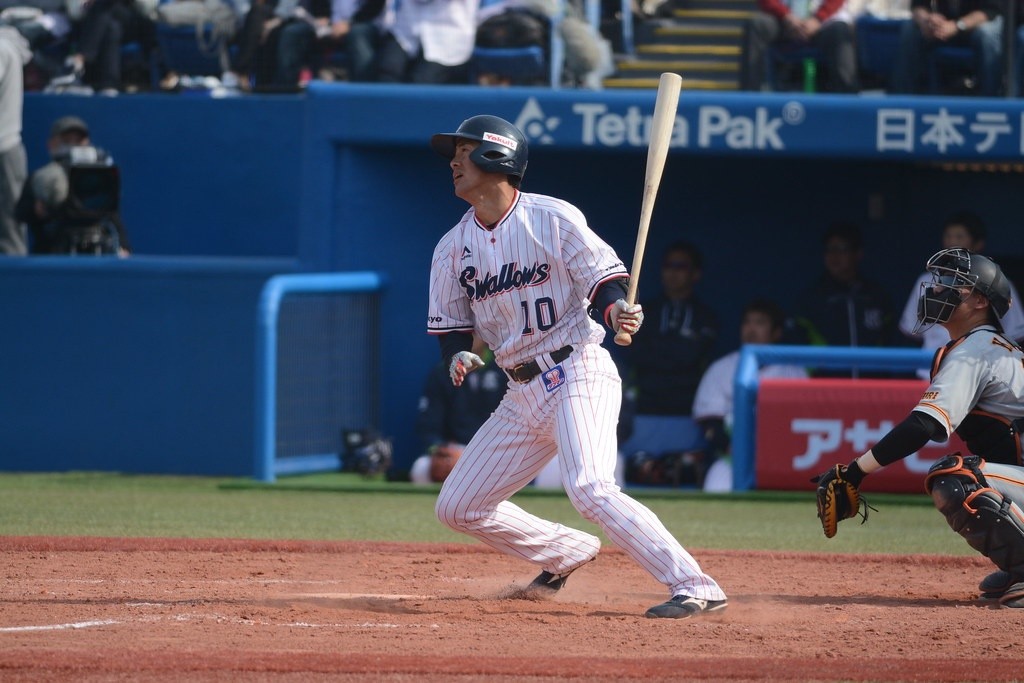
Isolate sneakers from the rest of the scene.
[978,570,1024,608]
[645,595,731,620]
[525,556,598,597]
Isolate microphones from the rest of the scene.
[29,162,70,205]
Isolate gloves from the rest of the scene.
[449,351,485,389]
[610,299,644,335]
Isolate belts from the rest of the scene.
[505,344,574,384]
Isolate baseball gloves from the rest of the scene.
[810,464,861,539]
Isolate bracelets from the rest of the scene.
[956,19,965,31]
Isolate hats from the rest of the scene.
[50,116,91,137]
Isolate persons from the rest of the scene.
[14,113,132,259]
[0,4,28,256]
[64,0,480,94]
[424,114,729,620]
[810,247,1023,609]
[409,213,1024,495]
[756,0,1008,97]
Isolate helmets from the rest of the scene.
[432,115,529,183]
[937,247,1011,334]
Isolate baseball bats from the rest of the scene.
[614,71,683,348]
